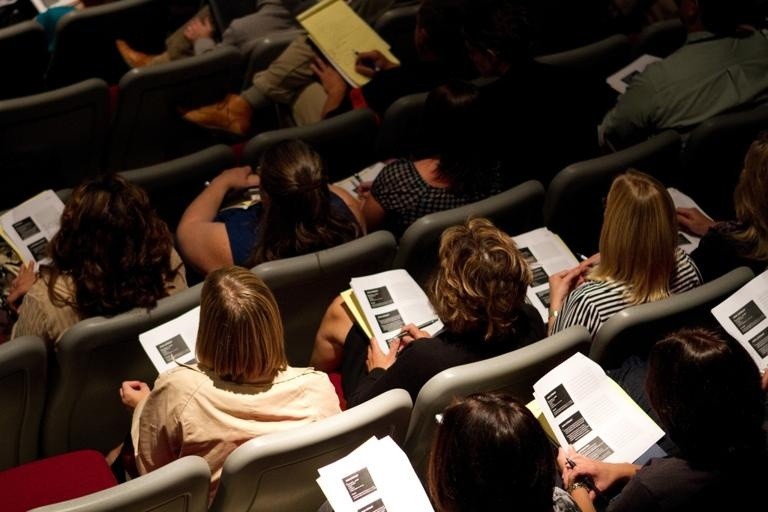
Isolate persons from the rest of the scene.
[420,386,557,511]
[27,1,584,149]
[551,165,712,341]
[557,325,767,511]
[172,133,371,278]
[352,78,532,247]
[585,1,768,150]
[4,160,187,344]
[673,135,768,281]
[110,265,348,511]
[339,216,550,410]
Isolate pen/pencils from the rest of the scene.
[351,48,380,72]
[354,172,363,184]
[566,457,601,495]
[396,319,438,338]
[574,252,589,261]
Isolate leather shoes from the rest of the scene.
[117,40,151,68]
[176,94,252,134]
